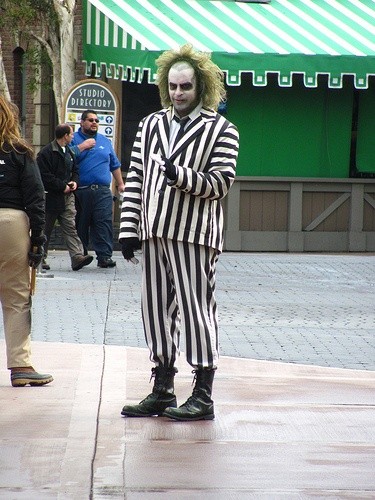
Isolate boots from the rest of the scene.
[163,366,218,420]
[121,366,178,416]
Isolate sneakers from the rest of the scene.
[10,367,54,386]
[98,258,116,268]
[42,259,50,269]
[71,255,93,270]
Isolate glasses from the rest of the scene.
[85,118,99,123]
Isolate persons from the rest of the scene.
[71,110,124,268]
[36,124,94,271]
[0,92,54,387]
[118,45,240,421]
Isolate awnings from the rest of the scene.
[85,0,375,89]
